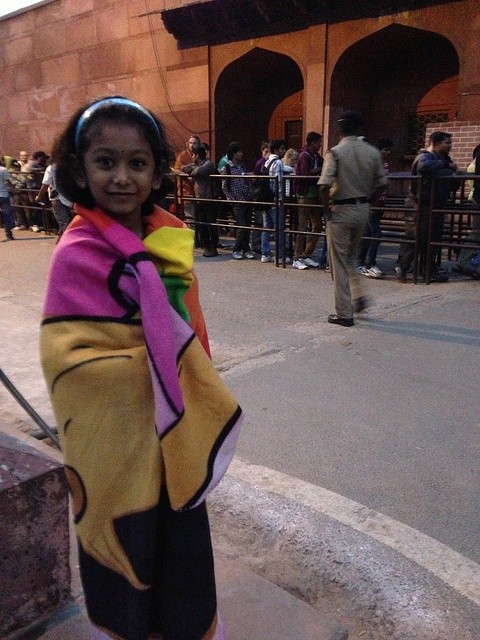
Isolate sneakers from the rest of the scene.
[260,254,273,263]
[232,251,242,259]
[357,266,383,278]
[303,257,320,268]
[274,256,291,264]
[292,258,308,270]
[244,250,255,259]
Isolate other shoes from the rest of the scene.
[392,263,406,281]
[202,248,218,257]
[427,273,448,283]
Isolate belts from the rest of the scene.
[332,197,370,205]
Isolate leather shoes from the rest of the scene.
[328,314,353,326]
[352,299,363,312]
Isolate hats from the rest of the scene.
[338,111,361,123]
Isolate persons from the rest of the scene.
[357,132,480,286]
[316,109,389,327]
[40,95,244,640]
[172,130,332,273]
[0,151,70,245]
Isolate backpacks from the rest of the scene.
[252,158,278,210]
